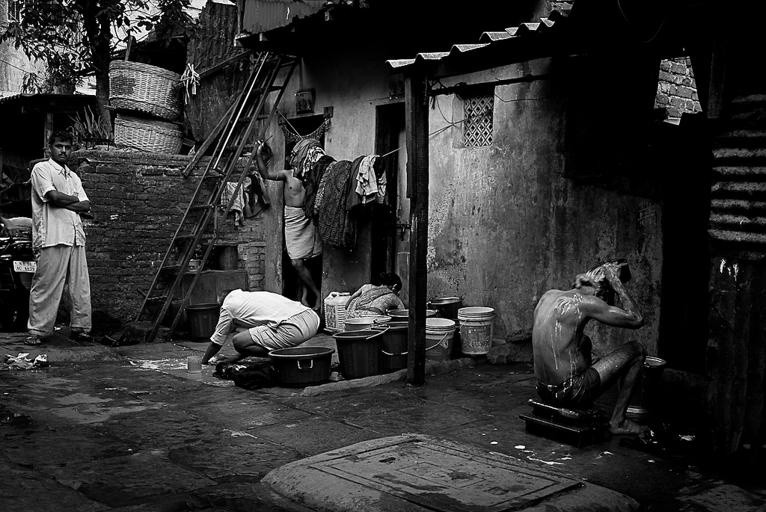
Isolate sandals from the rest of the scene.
[68,329,98,343]
[23,332,47,347]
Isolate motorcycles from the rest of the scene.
[0,223,37,332]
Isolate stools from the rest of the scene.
[518,397,599,447]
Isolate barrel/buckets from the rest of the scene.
[457,306,495,355]
[625,356,665,415]
[324,292,461,379]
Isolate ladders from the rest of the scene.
[134,47,300,346]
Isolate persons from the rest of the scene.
[20,130,98,347]
[200,287,323,366]
[254,139,325,313]
[527,264,647,439]
[345,272,405,319]
